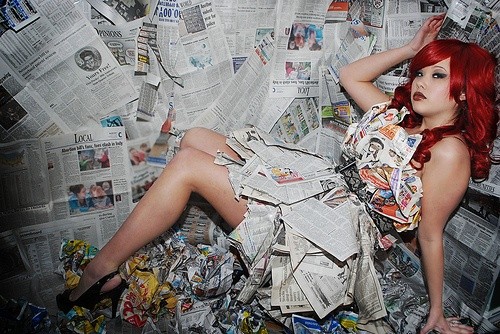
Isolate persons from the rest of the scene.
[53,11,500,333]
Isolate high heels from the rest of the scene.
[56,270,127,318]
